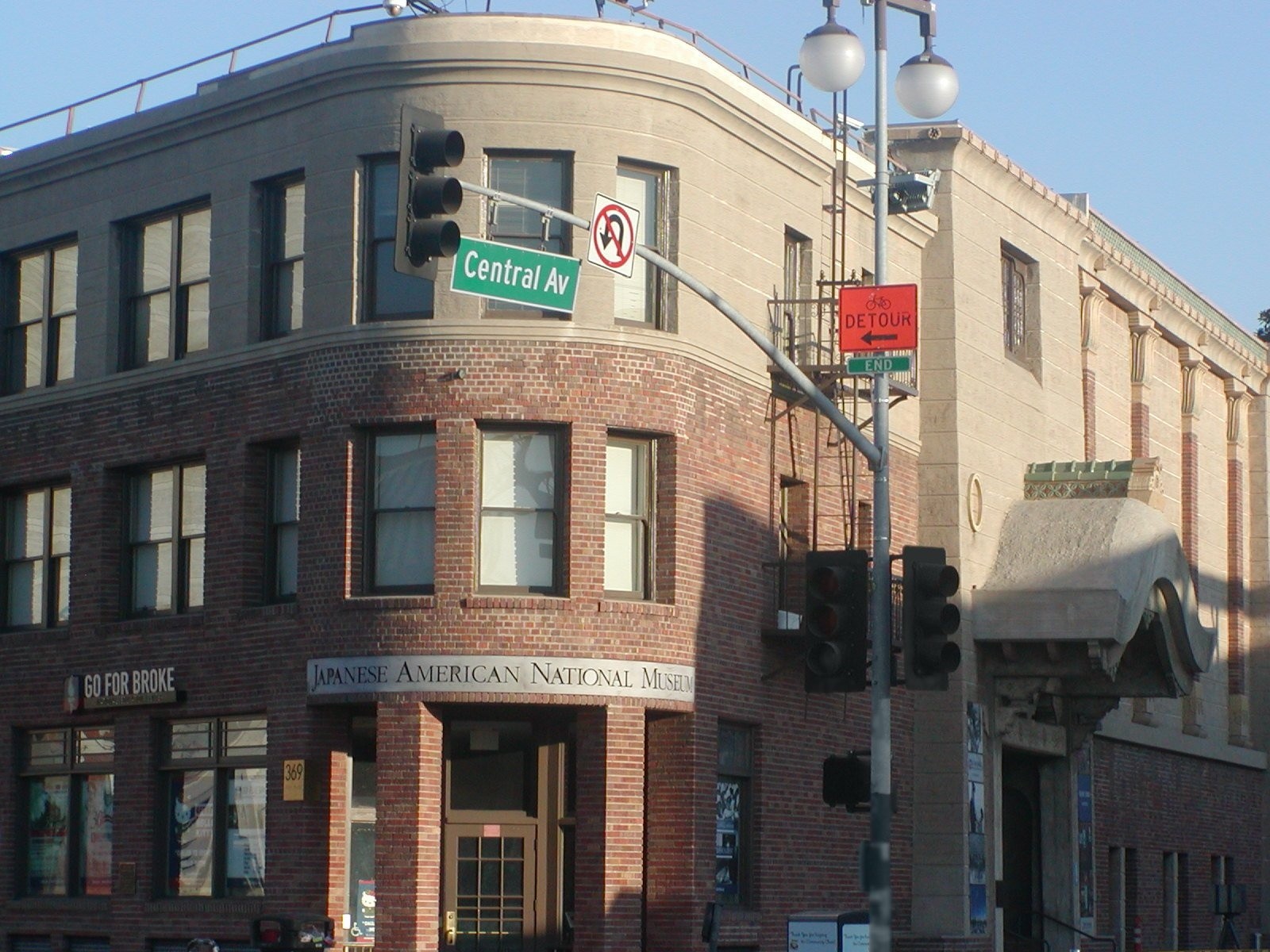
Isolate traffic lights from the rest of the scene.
[823,752,868,804]
[393,103,465,282]
[805,549,868,692]
[902,545,961,691]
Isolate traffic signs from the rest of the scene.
[839,284,918,351]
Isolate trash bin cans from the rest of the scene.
[787,908,870,952]
[249,913,335,952]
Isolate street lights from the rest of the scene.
[798,0,959,952]
